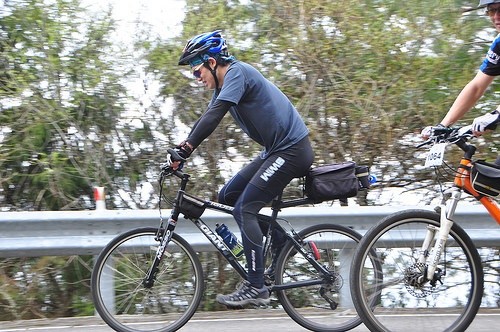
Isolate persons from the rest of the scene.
[162,28,315,307]
[420,0,500,197]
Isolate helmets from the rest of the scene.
[178,30,228,65]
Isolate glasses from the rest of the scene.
[193,62,204,77]
[485,7,500,16]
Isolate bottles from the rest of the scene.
[215,222,245,258]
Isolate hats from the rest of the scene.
[480,0,500,7]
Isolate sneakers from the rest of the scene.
[264,234,300,279]
[216,279,270,306]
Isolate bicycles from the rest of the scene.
[347,123,499,332]
[91,148,383,332]
